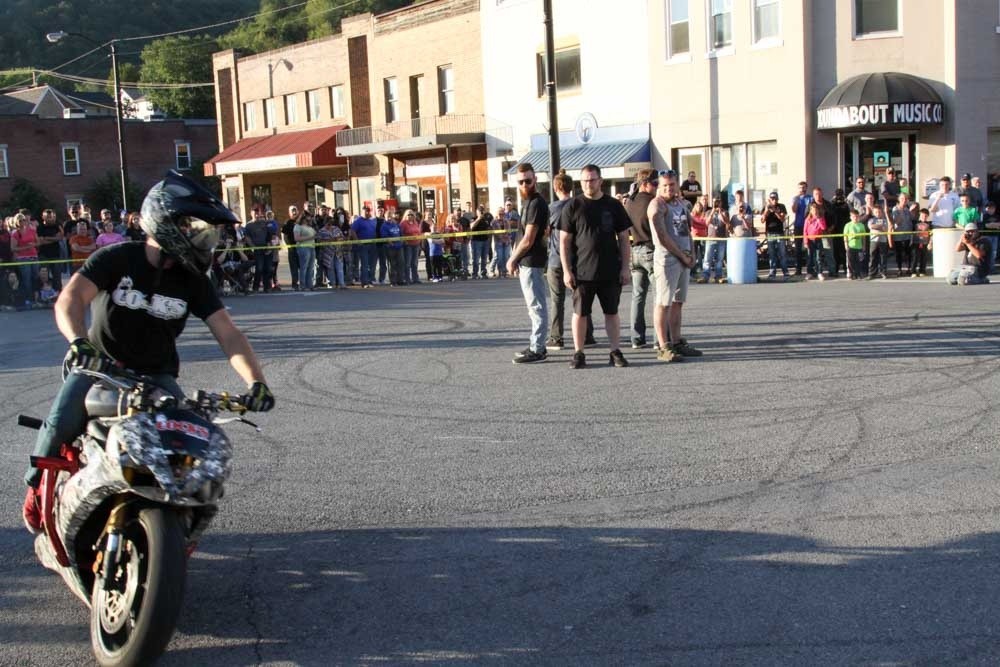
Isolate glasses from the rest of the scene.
[645,181,658,187]
[581,177,600,184]
[517,178,537,185]
[658,169,675,177]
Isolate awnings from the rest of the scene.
[818,71,943,133]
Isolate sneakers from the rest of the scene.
[569,350,585,369]
[515,348,548,357]
[545,336,565,350]
[657,342,683,361]
[512,351,547,364]
[22,486,46,535]
[631,336,647,348]
[583,336,598,347]
[609,349,626,367]
[673,338,703,356]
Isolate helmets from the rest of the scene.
[139,169,242,276]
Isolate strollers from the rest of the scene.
[434,244,469,282]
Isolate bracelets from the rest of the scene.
[773,211,775,214]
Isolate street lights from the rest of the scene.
[45,29,131,213]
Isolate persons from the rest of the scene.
[22,180,276,534]
[545,168,660,350]
[761,193,790,283]
[791,166,1000,284]
[647,170,702,361]
[555,164,628,369]
[681,171,752,283]
[281,201,520,291]
[506,162,550,365]
[0,203,282,314]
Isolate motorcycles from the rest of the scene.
[17,351,276,667]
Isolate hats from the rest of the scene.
[886,167,895,173]
[964,223,978,232]
[961,173,971,180]
[769,192,778,199]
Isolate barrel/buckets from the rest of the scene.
[931,228,967,277]
[726,239,759,284]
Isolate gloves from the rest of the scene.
[245,382,274,412]
[68,335,100,371]
